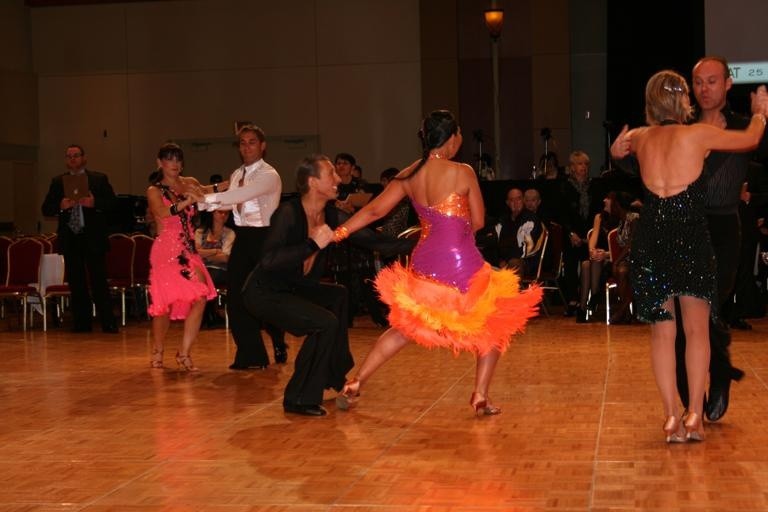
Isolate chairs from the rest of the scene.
[0,231,230,332]
[397,225,636,326]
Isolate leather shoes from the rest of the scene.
[331,376,360,398]
[705,374,732,421]
[284,406,327,417]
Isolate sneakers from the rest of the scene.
[273,344,288,365]
[575,294,601,324]
[229,360,267,371]
[71,317,119,336]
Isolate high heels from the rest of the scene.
[150,347,165,368]
[663,414,688,446]
[470,390,502,418]
[174,350,199,373]
[335,378,361,413]
[683,412,706,443]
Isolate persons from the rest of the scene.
[146,124,288,375]
[41,145,119,333]
[609,58,768,442]
[490,149,643,325]
[256,109,545,416]
